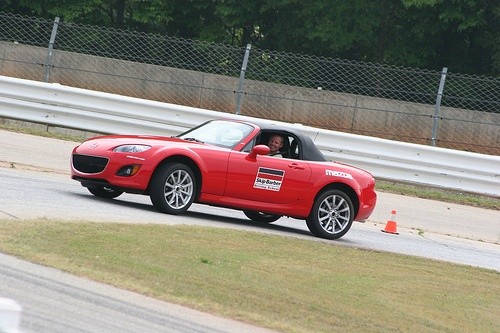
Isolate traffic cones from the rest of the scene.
[381,209,399,235]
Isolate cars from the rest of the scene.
[70,117,376,241]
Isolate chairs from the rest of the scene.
[281,138,291,158]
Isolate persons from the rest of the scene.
[265,134,285,159]
